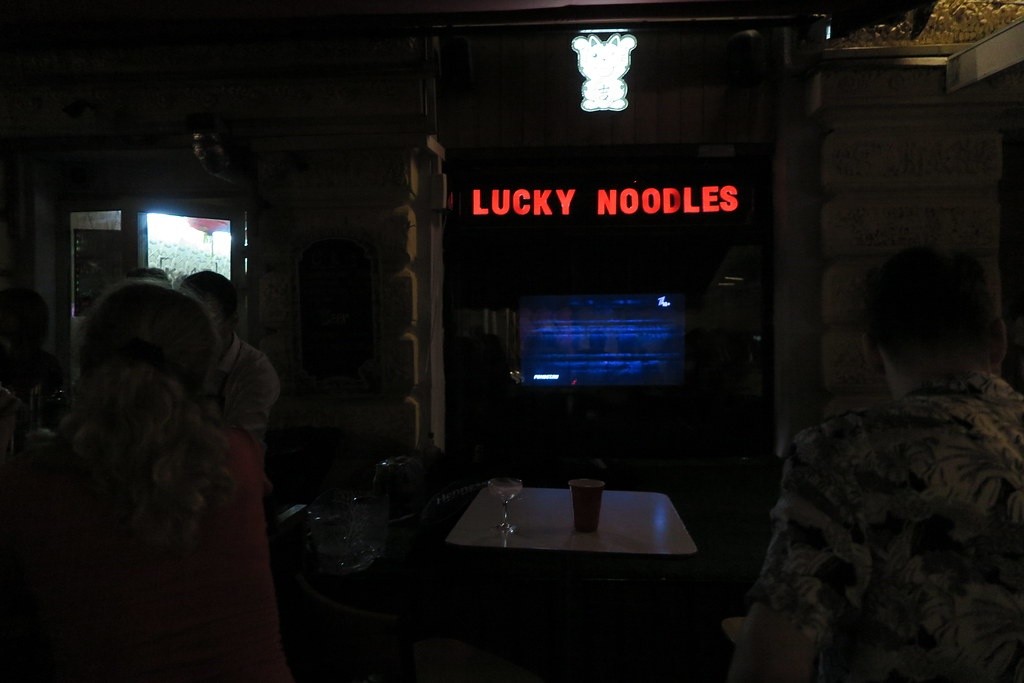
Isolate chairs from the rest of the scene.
[296,576,544,683]
[265,425,344,572]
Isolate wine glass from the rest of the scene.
[487,478,524,536]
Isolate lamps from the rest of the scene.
[807,8,833,41]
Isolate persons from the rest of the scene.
[179,271,282,439]
[0,273,297,683]
[726,243,1024,683]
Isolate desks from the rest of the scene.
[446,482,700,683]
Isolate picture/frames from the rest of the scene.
[289,227,384,393]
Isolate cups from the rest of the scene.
[568,479,605,533]
